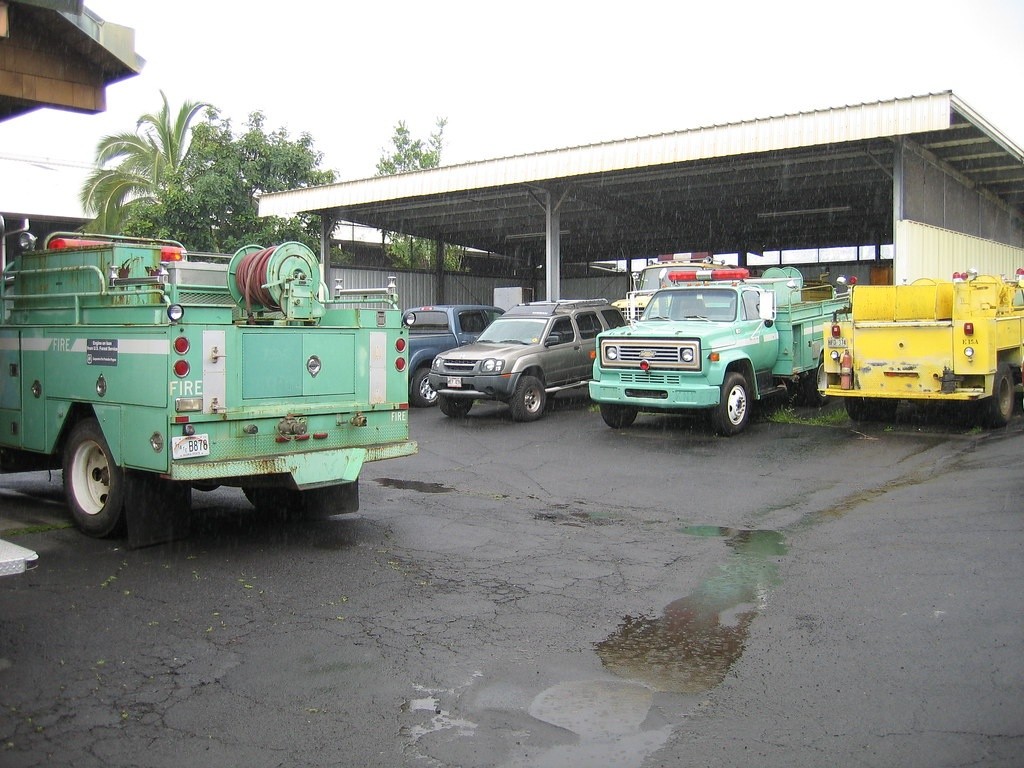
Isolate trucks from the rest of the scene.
[612,258,840,325]
[821,271,1024,428]
[587,267,853,436]
[401,305,509,407]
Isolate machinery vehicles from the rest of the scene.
[1,231,419,549]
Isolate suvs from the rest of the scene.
[435,298,627,422]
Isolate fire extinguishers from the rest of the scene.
[839,348,853,390]
